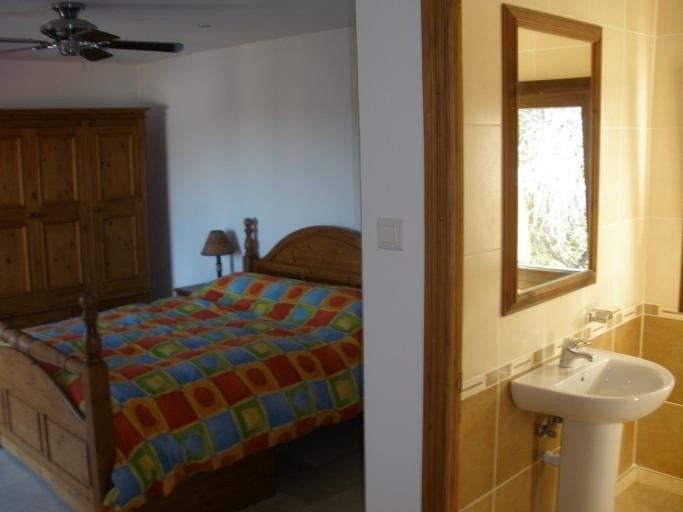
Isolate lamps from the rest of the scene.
[202,230,233,280]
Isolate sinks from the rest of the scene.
[512,347,675,421]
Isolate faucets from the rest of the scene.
[559,336,596,365]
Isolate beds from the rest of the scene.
[0,217,363,511]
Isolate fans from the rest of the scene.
[0,0,186,60]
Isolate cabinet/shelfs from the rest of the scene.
[0,105,155,327]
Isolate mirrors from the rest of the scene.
[501,4,597,318]
